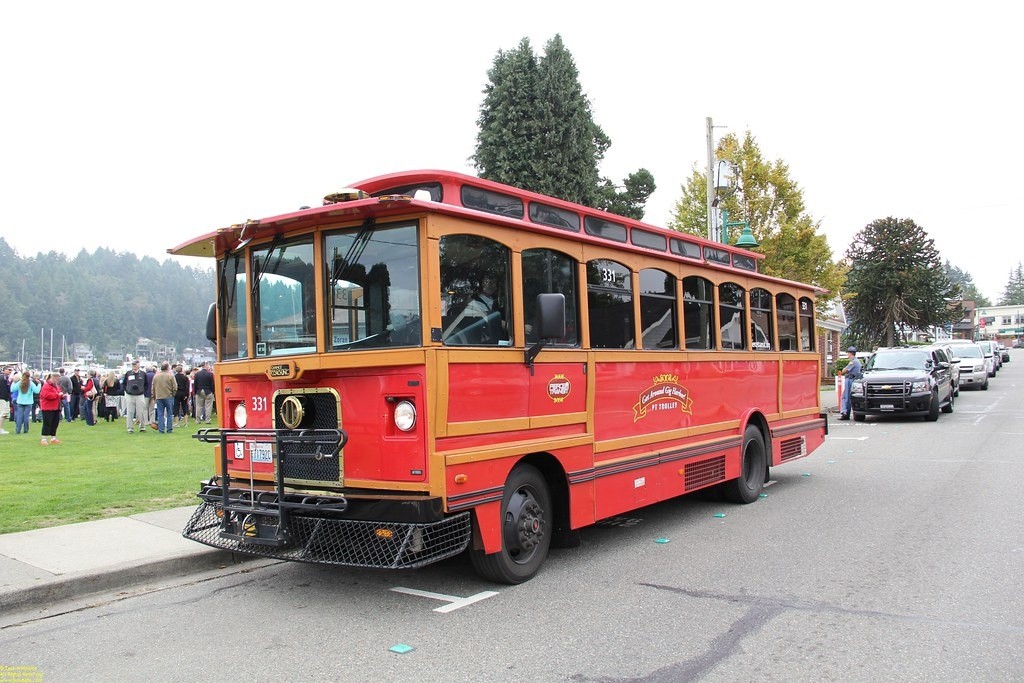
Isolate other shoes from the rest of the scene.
[183,423,187,427]
[129,431,134,434]
[71,420,74,423]
[140,430,148,433]
[0,428,9,434]
[32,420,36,423]
[837,414,850,420]
[36,419,42,422]
[172,423,180,427]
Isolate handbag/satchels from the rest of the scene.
[84,378,97,401]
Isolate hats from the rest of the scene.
[74,369,80,371]
[131,360,140,364]
[847,346,856,351]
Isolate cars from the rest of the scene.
[932,340,1009,378]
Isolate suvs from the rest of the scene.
[849,347,954,421]
[945,344,989,391]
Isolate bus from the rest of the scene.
[168,170,828,585]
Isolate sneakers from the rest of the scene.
[41,440,48,446]
[49,438,62,444]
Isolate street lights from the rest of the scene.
[723,219,760,251]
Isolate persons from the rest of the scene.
[40,372,63,446]
[10,372,41,433]
[0,366,10,434]
[194,362,215,424]
[151,361,178,433]
[838,346,861,420]
[490,274,538,342]
[122,360,149,433]
[58,368,74,423]
[173,366,190,428]
[102,369,121,422]
[0,362,215,426]
